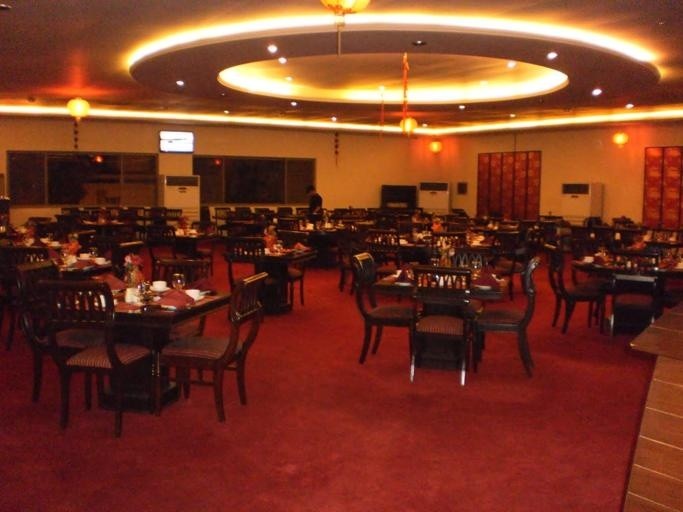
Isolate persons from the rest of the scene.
[302,184,324,220]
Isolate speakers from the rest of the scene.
[457,182,467,195]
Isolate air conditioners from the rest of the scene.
[160,174,201,220]
[560,181,603,217]
[417,182,451,214]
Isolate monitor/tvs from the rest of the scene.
[158,130,195,155]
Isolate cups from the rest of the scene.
[0,226,200,303]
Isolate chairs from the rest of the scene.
[159,271,269,423]
[609,248,662,336]
[4,243,51,350]
[157,256,211,381]
[352,253,417,364]
[38,278,158,437]
[471,253,541,378]
[3,200,681,313]
[15,260,109,408]
[407,264,472,386]
[544,243,605,333]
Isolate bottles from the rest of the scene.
[374,234,398,246]
[455,253,481,268]
[417,272,467,290]
[56,293,105,322]
[234,242,260,256]
[616,254,656,270]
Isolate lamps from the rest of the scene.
[399,115,419,141]
[321,0,370,57]
[67,97,91,122]
